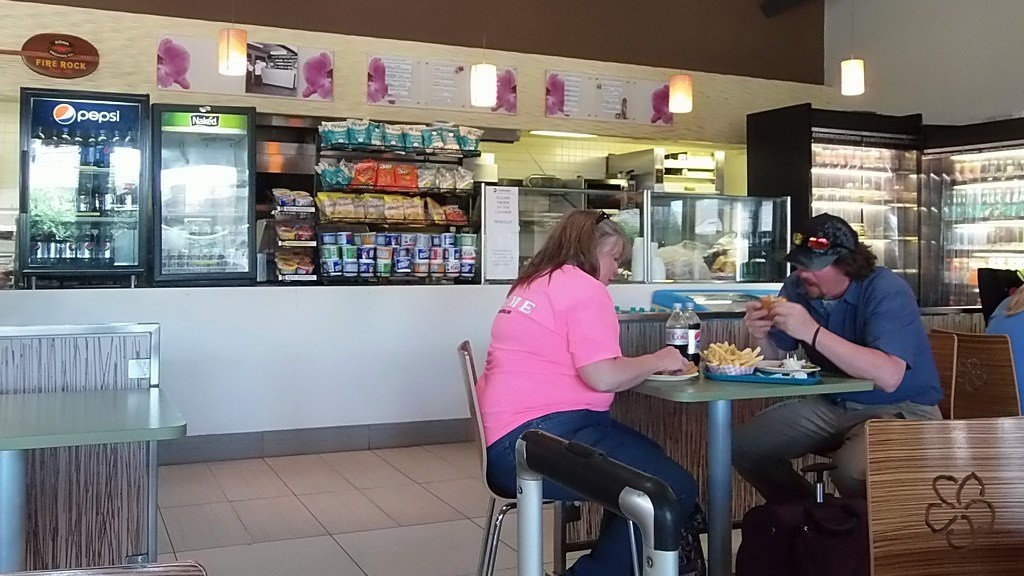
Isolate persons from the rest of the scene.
[476,209,699,576]
[730,212,944,506]
[984,284,1024,415]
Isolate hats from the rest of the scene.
[780,212,859,271]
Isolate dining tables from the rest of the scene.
[632,370,873,576]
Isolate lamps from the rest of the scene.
[470,33,497,107]
[218,16,247,76]
[840,0,865,96]
[669,74,693,113]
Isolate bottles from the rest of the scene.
[31,127,134,266]
[683,302,701,366]
[666,303,689,358]
[945,185,1024,219]
[321,232,478,277]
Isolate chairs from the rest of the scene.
[930,328,1020,421]
[811,334,957,457]
[458,341,607,576]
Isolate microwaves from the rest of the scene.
[550,178,635,213]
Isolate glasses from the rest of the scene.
[596,210,610,225]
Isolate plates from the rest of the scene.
[646,372,699,380]
[756,361,821,373]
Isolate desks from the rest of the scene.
[0,387,187,573]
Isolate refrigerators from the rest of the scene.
[651,290,780,312]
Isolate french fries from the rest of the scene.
[698,341,764,368]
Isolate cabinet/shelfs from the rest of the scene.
[518,186,643,270]
[313,134,481,285]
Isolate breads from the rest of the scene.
[684,361,699,374]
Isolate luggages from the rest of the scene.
[735,462,870,575]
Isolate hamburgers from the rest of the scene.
[755,294,787,320]
